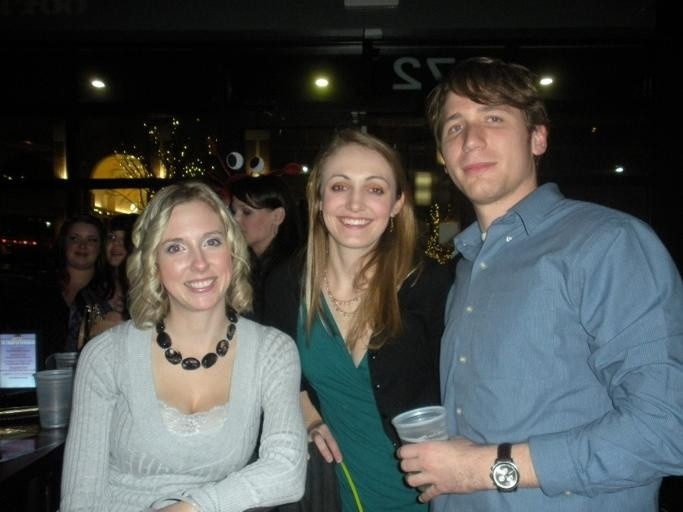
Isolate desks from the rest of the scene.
[0,405,72,512]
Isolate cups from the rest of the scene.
[390,405,449,496]
[31,367,73,430]
[50,351,80,369]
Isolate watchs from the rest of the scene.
[488,442,522,493]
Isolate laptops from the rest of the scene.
[0,330,39,416]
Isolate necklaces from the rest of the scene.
[149,302,240,376]
[321,252,370,319]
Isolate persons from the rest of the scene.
[94,212,134,325]
[31,211,109,362]
[226,172,308,334]
[54,182,310,512]
[394,54,683,512]
[258,126,453,512]
